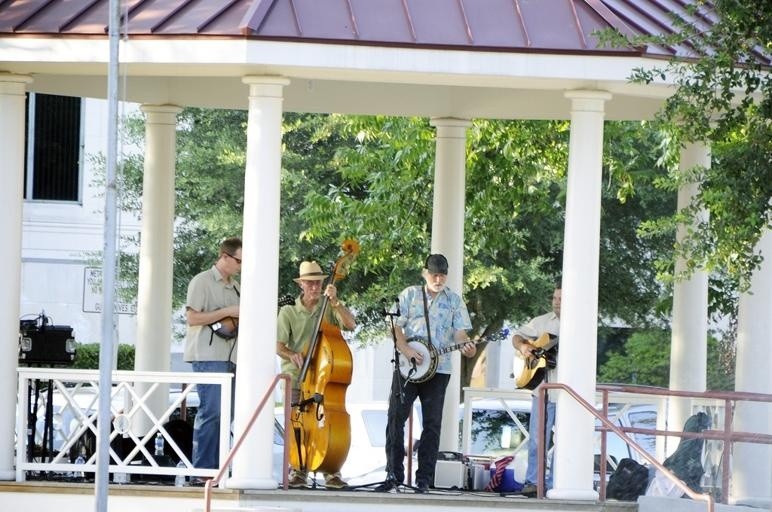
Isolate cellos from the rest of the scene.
[288,239,358,475]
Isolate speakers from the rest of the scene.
[24,93,84,207]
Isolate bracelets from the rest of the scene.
[330,299,340,308]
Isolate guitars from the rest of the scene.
[208,294,301,338]
[513,333,559,390]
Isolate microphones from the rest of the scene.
[381,298,386,316]
[528,348,540,357]
[395,298,400,317]
[410,357,417,372]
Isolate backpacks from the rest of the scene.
[606,458,649,501]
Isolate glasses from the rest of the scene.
[225,252,241,263]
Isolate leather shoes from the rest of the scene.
[376,481,402,492]
[415,483,429,493]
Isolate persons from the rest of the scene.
[511,285,563,496]
[183,237,243,488]
[276,260,358,491]
[372,253,478,493]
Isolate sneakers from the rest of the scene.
[279,475,307,487]
[324,476,348,489]
[522,482,548,495]
[190,477,218,487]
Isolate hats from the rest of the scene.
[425,255,449,275]
[292,261,329,284]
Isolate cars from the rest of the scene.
[170,347,739,504]
